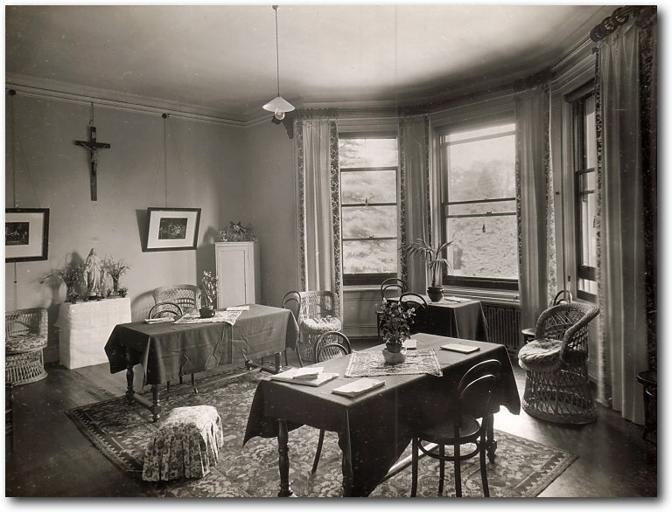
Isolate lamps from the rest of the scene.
[262,5,294,120]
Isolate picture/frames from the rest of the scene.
[142,207,201,251]
[5,208,50,263]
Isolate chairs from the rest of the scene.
[519,301,602,426]
[310,329,354,475]
[153,283,196,315]
[410,358,501,497]
[149,302,186,392]
[376,279,407,337]
[522,290,574,351]
[281,290,346,366]
[5,308,49,388]
[399,292,441,336]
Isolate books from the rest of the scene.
[332,377,385,397]
[384,338,417,350]
[270,366,339,387]
[444,296,471,303]
[225,305,250,311]
[440,343,481,354]
[144,316,176,325]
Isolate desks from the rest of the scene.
[242,332,520,497]
[59,297,132,370]
[105,302,293,422]
[387,292,489,337]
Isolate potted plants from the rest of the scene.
[406,241,455,302]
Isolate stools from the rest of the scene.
[141,404,223,481]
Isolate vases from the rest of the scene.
[63,280,82,303]
[382,345,407,365]
[107,275,122,298]
[200,303,214,318]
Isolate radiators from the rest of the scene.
[484,297,533,363]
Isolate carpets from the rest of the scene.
[64,359,580,496]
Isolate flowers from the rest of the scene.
[199,271,218,317]
[379,278,419,347]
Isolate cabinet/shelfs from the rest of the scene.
[215,240,262,309]
